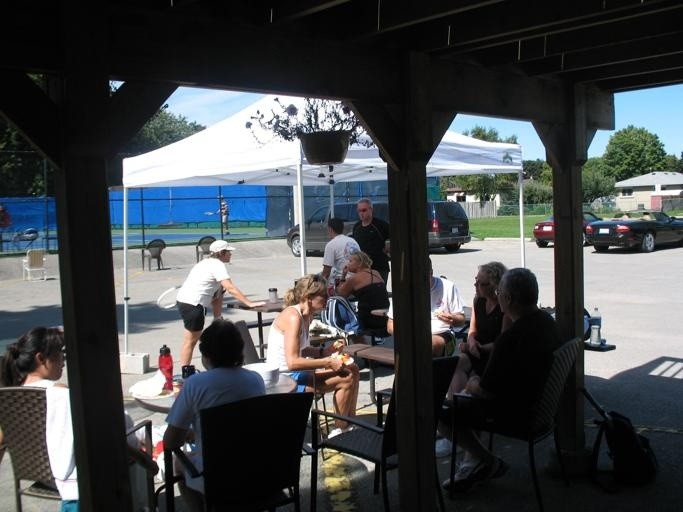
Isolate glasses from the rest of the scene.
[474,276,491,287]
[306,274,320,290]
[494,288,506,297]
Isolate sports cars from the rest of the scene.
[585,209,682,253]
[532,211,604,247]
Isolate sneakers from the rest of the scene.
[327,425,359,440]
[441,455,507,491]
[374,336,387,346]
[434,436,465,459]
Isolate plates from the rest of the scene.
[130,383,176,399]
[332,352,354,365]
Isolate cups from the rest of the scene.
[181,365,196,379]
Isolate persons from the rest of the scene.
[217,203,230,237]
[385,258,466,360]
[157,316,267,512]
[438,267,555,495]
[267,273,359,440]
[1,325,154,503]
[336,251,390,329]
[321,218,361,287]
[353,199,393,286]
[443,262,508,400]
[174,239,267,377]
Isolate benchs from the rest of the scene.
[341,342,394,401]
[247,317,274,331]
[251,334,341,349]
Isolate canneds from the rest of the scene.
[269,288,278,303]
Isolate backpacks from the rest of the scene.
[587,407,658,493]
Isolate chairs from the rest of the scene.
[195,236,217,263]
[23,249,47,282]
[141,239,166,270]
[450,336,582,512]
[156,391,314,511]
[0,386,159,511]
[311,356,462,512]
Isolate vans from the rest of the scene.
[424,200,472,254]
[286,202,396,259]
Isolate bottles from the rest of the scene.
[158,343,173,393]
[590,306,602,348]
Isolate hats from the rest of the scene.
[207,239,236,254]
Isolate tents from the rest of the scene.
[122,94,527,358]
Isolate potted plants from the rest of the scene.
[242,98,376,166]
